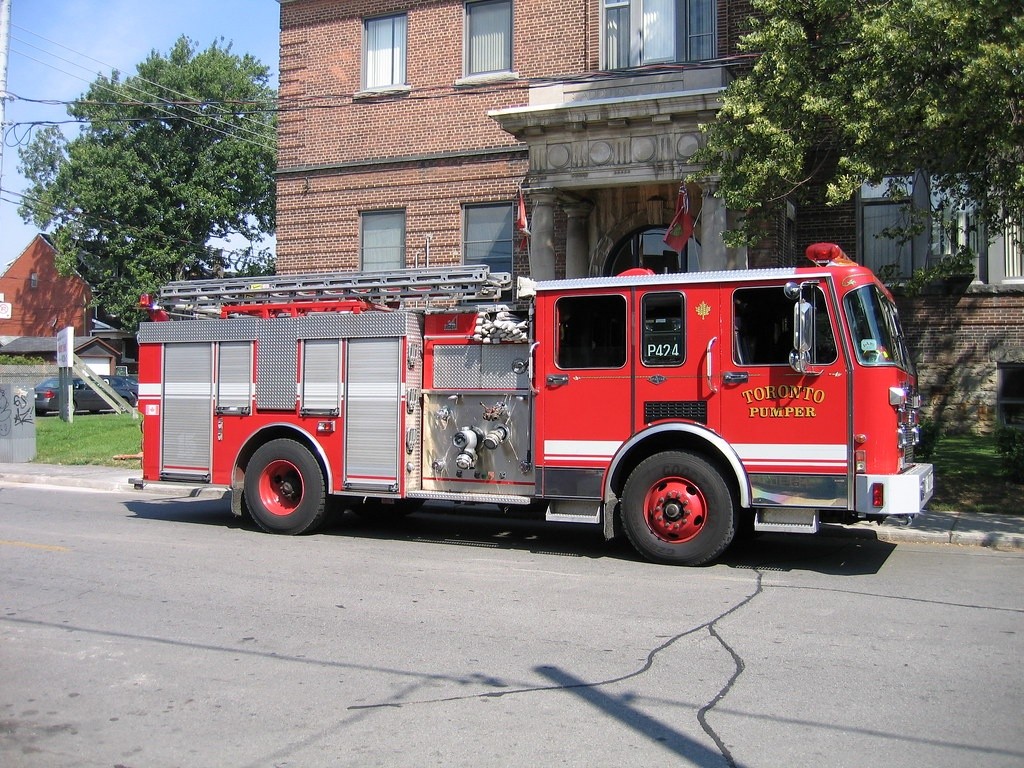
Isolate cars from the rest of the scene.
[34,377,138,415]
[86,375,138,401]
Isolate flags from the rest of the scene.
[663,175,693,254]
[517,186,531,252]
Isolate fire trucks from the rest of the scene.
[127,242,933,568]
